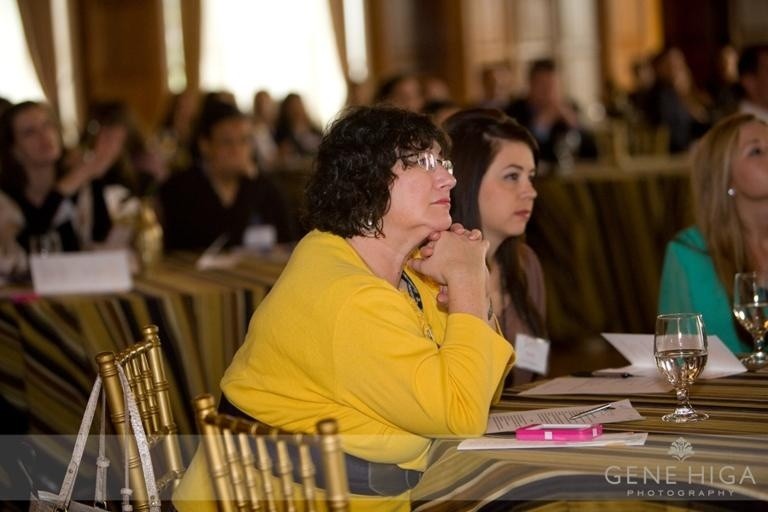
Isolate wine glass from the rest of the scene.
[733,272,768,368]
[653,312,711,422]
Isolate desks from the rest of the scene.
[432,354,767,510]
[2,241,304,512]
[534,154,694,364]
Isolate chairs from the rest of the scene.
[94,320,353,511]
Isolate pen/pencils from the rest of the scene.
[573,372,633,378]
[570,404,610,419]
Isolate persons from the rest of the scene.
[175,100,549,510]
[0,38,768,279]
[658,109,768,359]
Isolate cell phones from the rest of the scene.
[515,423,604,441]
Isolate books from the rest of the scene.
[460,399,650,450]
[522,333,747,396]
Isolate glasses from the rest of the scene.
[393,150,455,178]
